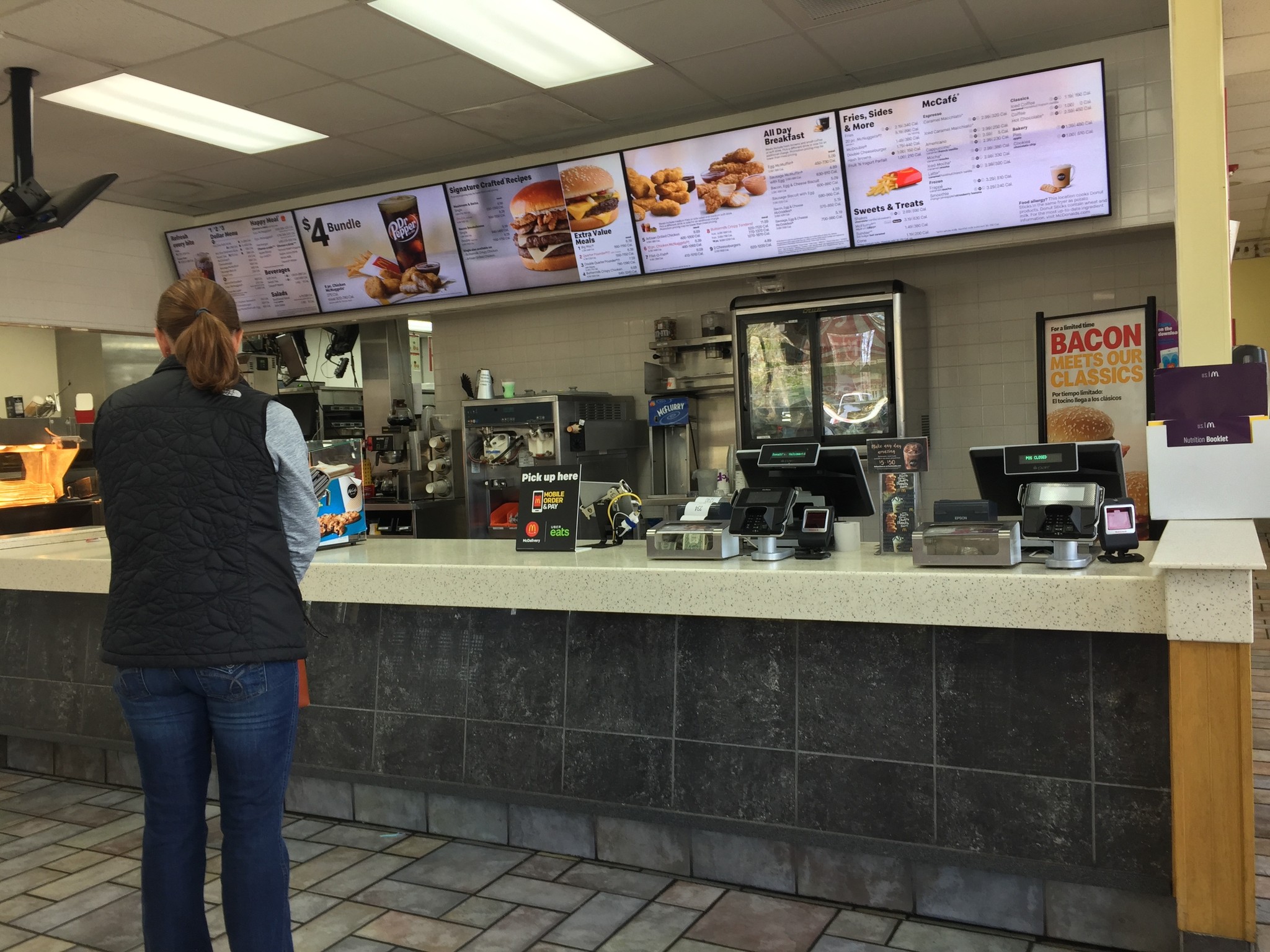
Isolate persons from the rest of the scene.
[91,278,321,952]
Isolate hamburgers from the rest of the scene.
[509,179,578,271]
[559,164,619,232]
[1046,405,1131,459]
[1123,470,1151,540]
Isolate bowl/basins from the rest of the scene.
[51,436,80,449]
[415,262,440,276]
[700,168,726,183]
[741,173,767,195]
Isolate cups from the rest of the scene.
[726,444,736,494]
[426,480,447,493]
[717,469,730,496]
[903,442,923,470]
[429,436,445,449]
[892,502,904,513]
[377,195,427,274]
[892,540,905,552]
[501,379,516,398]
[667,377,676,390]
[428,458,446,472]
[194,254,215,281]
[1051,164,1077,188]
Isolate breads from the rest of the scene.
[1040,183,1062,193]
[813,125,823,132]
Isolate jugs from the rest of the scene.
[387,399,414,426]
[381,469,399,496]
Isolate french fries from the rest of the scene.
[345,250,373,277]
[867,173,899,196]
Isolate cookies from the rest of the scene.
[317,511,361,537]
